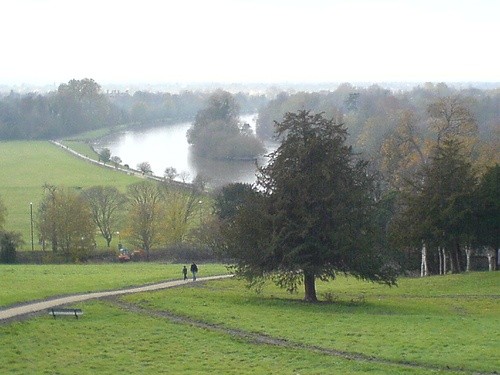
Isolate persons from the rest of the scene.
[182,266,188,281]
[190,262,198,281]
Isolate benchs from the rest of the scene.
[46,306,84,319]
